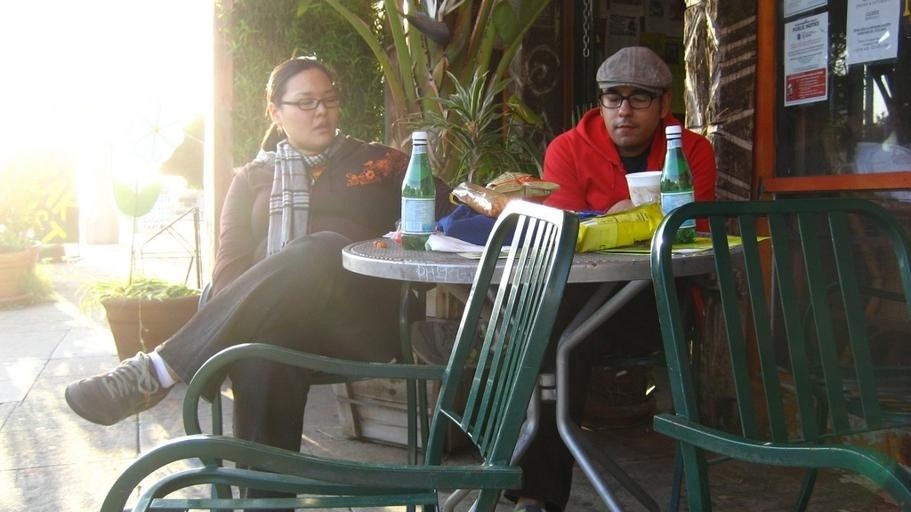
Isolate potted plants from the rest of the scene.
[294,1,562,456]
[0,167,73,311]
[81,276,202,363]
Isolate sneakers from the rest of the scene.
[411,318,509,370]
[66,351,175,425]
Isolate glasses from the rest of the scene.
[599,93,657,110]
[282,96,342,111]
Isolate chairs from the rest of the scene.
[795,283,909,510]
[670,276,746,509]
[99,196,580,511]
[648,196,910,510]
[212,280,440,511]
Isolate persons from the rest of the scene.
[826,88,911,205]
[503,47,719,511]
[64,56,458,511]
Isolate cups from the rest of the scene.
[624,169,661,207]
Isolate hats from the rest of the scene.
[597,47,673,95]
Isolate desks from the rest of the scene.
[342,230,764,510]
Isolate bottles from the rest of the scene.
[658,125,698,245]
[400,130,438,252]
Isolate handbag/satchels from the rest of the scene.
[435,204,604,245]
